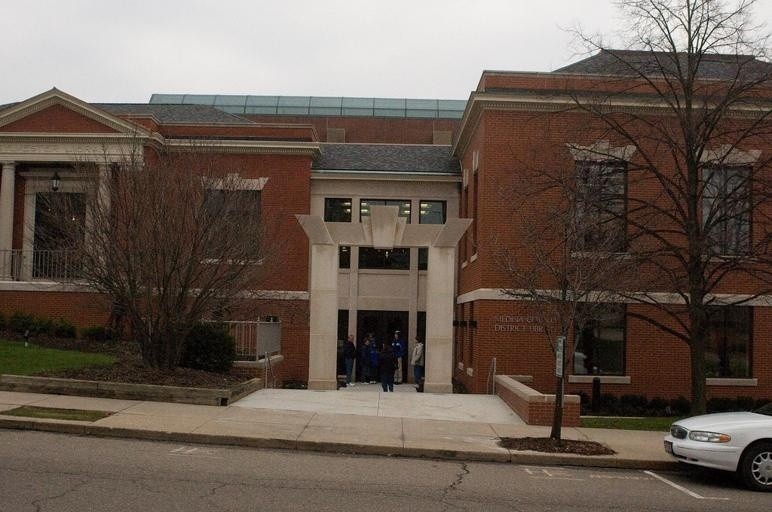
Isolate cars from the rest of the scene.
[662,396,772,492]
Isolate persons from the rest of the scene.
[409,334,425,392]
[342,328,407,393]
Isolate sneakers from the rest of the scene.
[346,381,419,388]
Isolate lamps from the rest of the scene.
[51,164,62,193]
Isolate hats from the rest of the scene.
[394,330,401,335]
